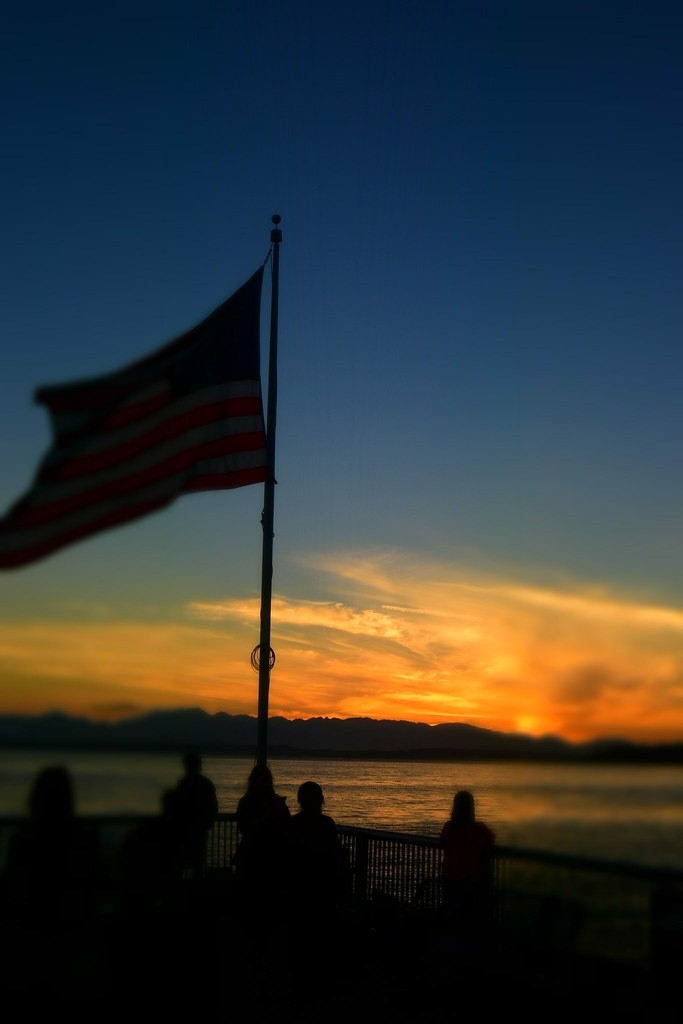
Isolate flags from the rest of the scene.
[1,264,269,574]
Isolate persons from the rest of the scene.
[0,754,350,900]
[437,789,497,929]
